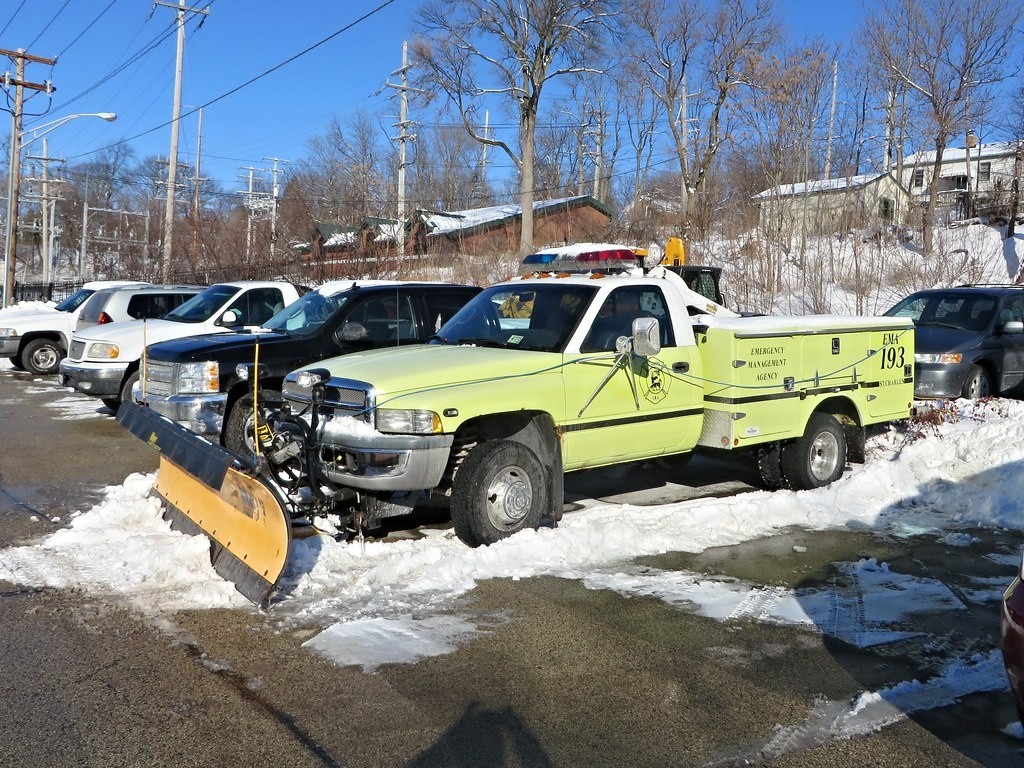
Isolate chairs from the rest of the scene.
[366,301,390,340]
[597,310,656,351]
[1000,308,1015,325]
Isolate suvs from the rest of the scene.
[0,280,210,377]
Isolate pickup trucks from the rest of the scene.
[135,278,533,474]
[59,279,411,412]
[116,247,918,605]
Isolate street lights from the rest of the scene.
[4,112,118,306]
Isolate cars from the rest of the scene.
[882,283,1024,402]
